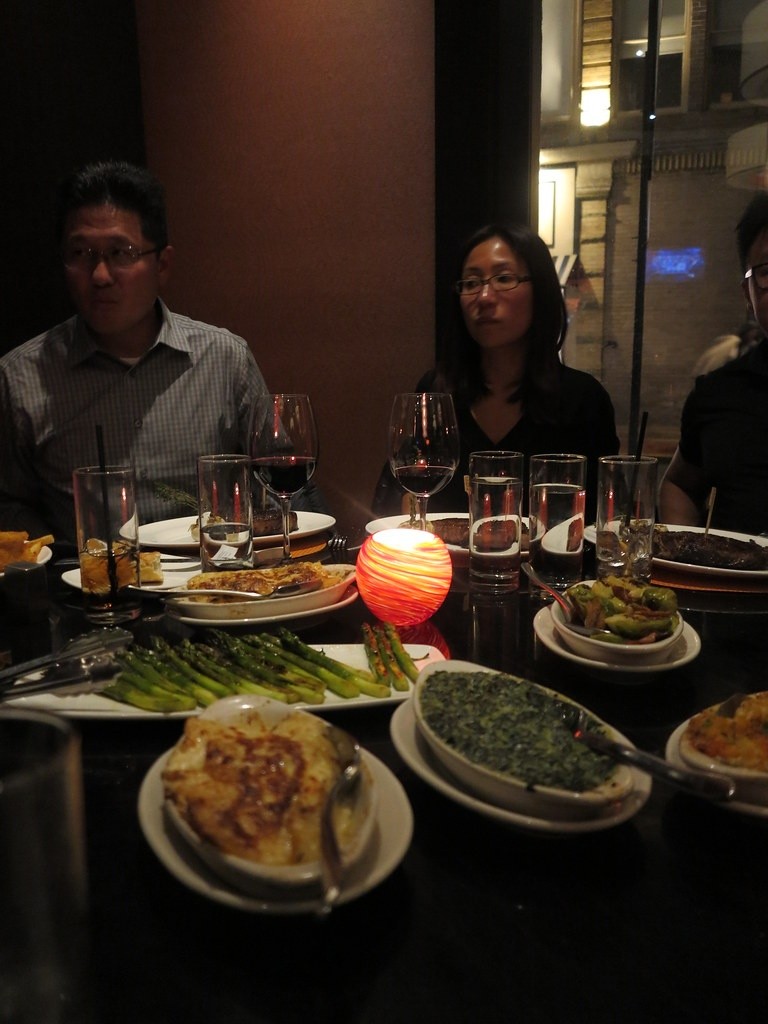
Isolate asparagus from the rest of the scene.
[94,622,421,712]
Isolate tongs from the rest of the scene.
[0,626,134,702]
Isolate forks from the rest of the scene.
[326,534,348,564]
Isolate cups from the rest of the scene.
[467,452,657,593]
[73,455,252,592]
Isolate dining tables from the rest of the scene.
[0,543,767,1024]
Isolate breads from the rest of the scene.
[80,545,163,592]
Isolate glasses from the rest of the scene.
[744,263,768,291]
[455,273,533,295]
[60,244,161,266]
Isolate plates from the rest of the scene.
[62,553,215,592]
[583,520,768,574]
[137,748,413,912]
[666,714,768,815]
[119,511,336,546]
[0,540,52,577]
[165,585,358,623]
[366,513,546,555]
[390,695,652,829]
[3,645,448,718]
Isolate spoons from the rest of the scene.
[581,733,735,798]
[126,579,323,599]
[323,725,362,913]
[520,561,610,636]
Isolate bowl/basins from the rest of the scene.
[551,580,684,665]
[534,604,701,671]
[413,660,634,820]
[160,563,356,620]
[162,693,375,884]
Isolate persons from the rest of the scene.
[656,191,768,537]
[372,223,621,526]
[0,160,325,539]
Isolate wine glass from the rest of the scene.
[390,393,461,533]
[247,393,318,563]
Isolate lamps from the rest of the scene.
[723,3,768,192]
